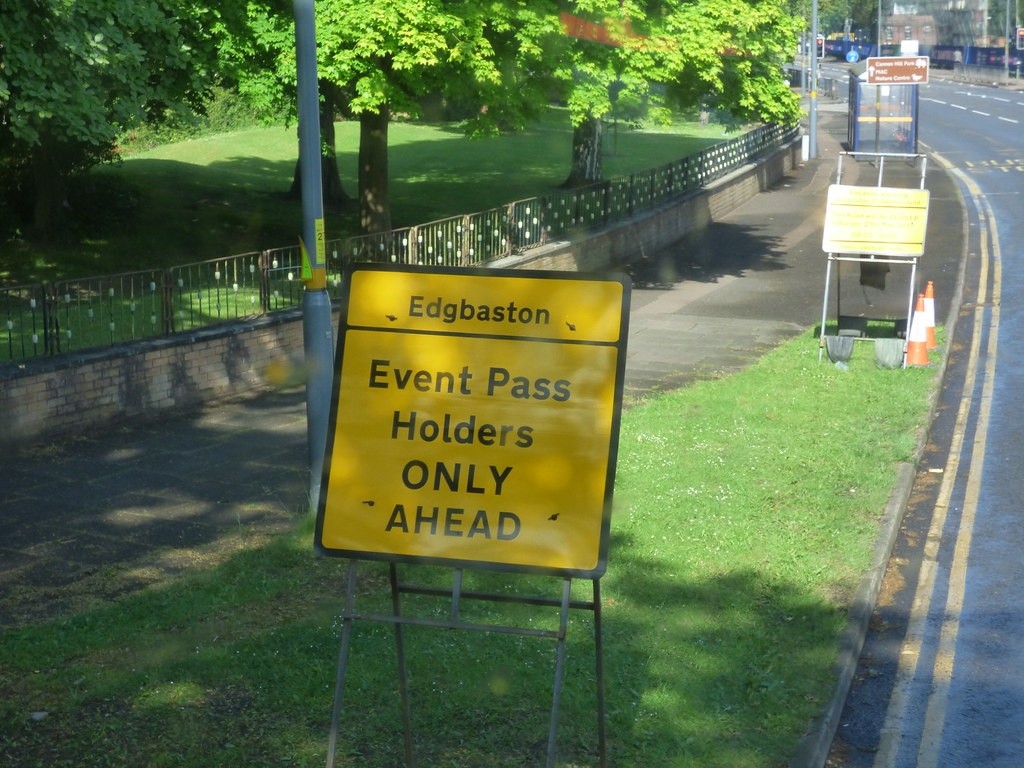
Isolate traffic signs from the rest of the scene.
[866,56,930,85]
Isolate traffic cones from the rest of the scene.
[923,279,942,350]
[906,293,935,367]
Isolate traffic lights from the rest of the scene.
[1016,28,1024,50]
